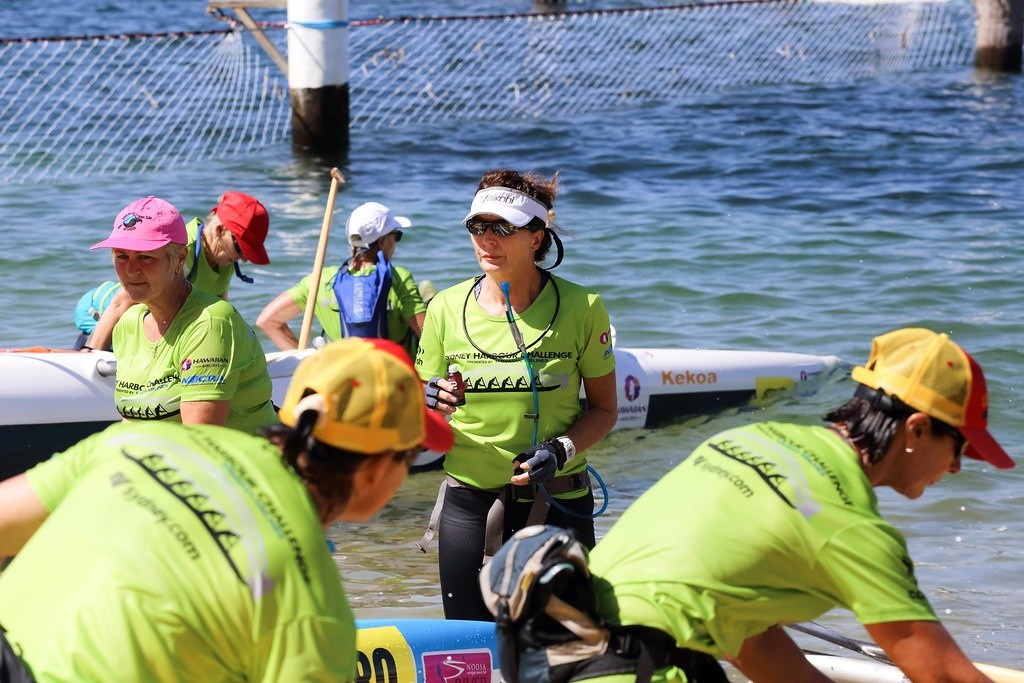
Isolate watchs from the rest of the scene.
[557,436,576,464]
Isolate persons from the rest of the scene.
[0,336,452,683]
[71,192,270,350]
[565,327,1014,683]
[413,170,619,622]
[256,202,439,362]
[90,196,280,436]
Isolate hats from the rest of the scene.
[90,196,188,252]
[461,186,548,228]
[345,202,411,249]
[851,328,1016,469]
[277,337,455,455]
[210,191,271,265]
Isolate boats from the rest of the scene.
[1,346,857,474]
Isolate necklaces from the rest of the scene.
[151,284,187,359]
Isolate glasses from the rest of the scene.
[932,422,969,459]
[388,230,403,243]
[231,235,250,263]
[465,216,530,237]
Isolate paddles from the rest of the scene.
[297,166,348,350]
